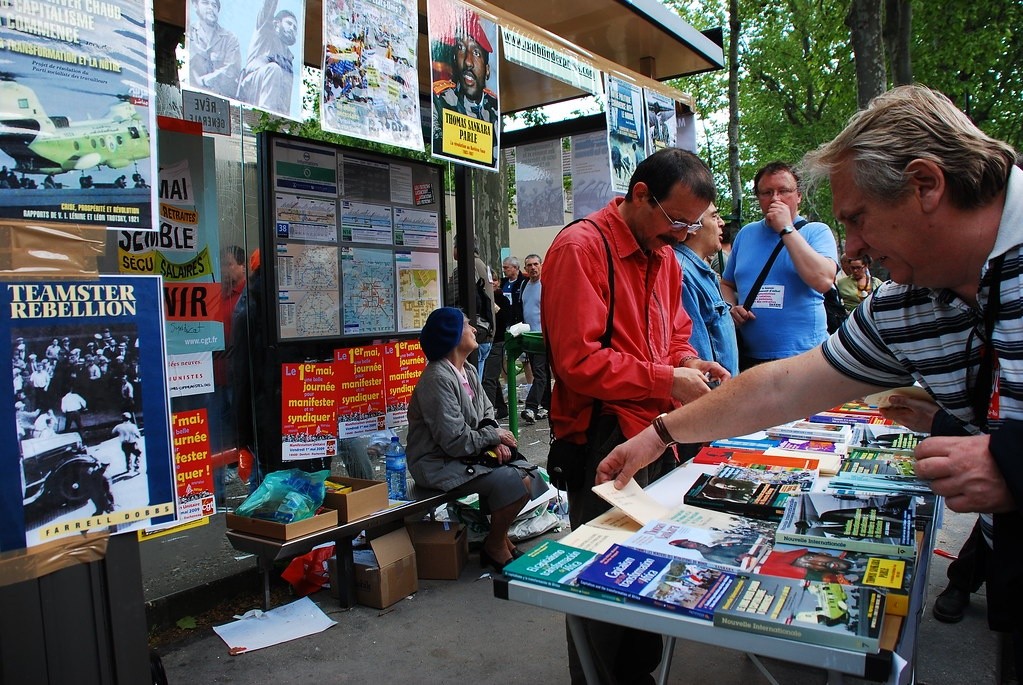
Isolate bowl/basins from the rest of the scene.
[505,330,543,350]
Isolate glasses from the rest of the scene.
[652,194,703,234]
[849,266,865,271]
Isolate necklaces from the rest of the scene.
[856,274,869,297]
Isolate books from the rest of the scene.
[503,399,936,654]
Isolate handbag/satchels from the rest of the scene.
[546,439,590,492]
[461,418,518,469]
[233,468,331,523]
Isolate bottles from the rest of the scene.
[386,437,407,499]
[330,451,350,478]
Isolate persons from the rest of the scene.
[187,0,242,97]
[720,161,838,373]
[632,144,642,167]
[785,549,869,585]
[877,394,992,625]
[404,308,550,573]
[13,328,142,514]
[596,84,1023,685]
[215,246,268,491]
[707,222,741,276]
[450,235,552,422]
[540,148,729,685]
[673,202,738,468]
[0,166,20,189]
[115,174,125,189]
[241,0,298,117]
[671,539,752,567]
[432,11,500,167]
[822,244,891,335]
[45,171,54,188]
[82,175,92,188]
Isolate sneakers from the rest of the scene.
[933,582,971,623]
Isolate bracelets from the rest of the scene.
[679,356,699,367]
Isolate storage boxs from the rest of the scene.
[365,511,467,581]
[324,476,389,523]
[320,527,418,609]
[225,508,338,540]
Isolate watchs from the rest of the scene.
[779,225,793,238]
[651,412,678,447]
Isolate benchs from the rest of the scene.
[225,483,477,613]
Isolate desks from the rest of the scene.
[508,455,943,685]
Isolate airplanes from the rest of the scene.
[0,70,150,186]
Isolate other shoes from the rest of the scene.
[495,413,508,419]
[534,408,549,421]
[521,409,537,423]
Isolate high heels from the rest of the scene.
[479,537,514,573]
[508,544,524,560]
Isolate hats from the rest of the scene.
[419,307,464,363]
